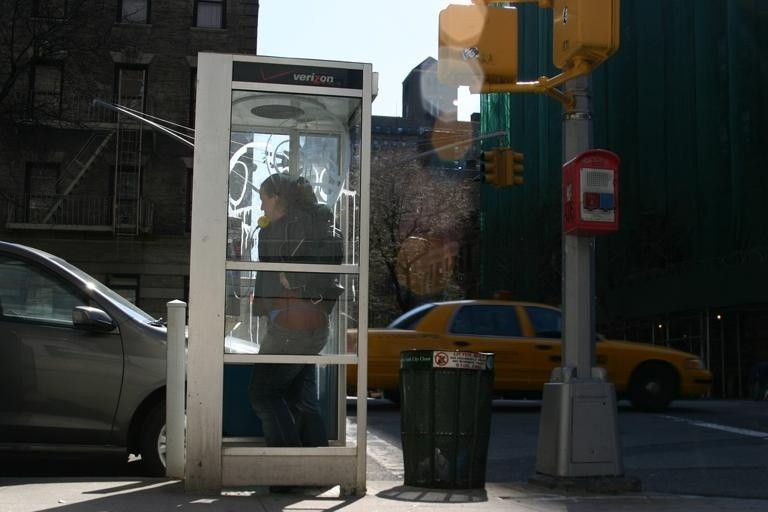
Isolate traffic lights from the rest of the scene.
[505,147,525,188]
[481,145,501,188]
[552,0,621,68]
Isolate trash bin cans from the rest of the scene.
[399,348,495,488]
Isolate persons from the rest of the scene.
[248,171,345,495]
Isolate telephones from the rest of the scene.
[258,215,271,228]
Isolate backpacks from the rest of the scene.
[300,203,345,314]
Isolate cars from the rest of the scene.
[0,242,259,472]
[345,292,715,411]
